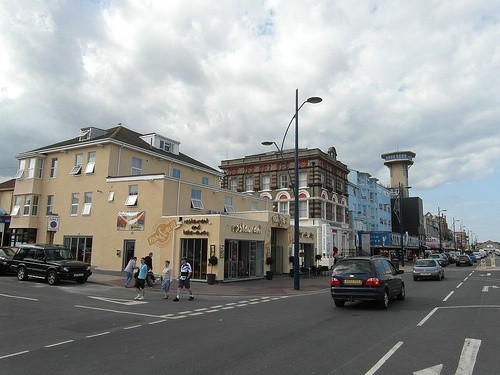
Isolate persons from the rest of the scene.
[333,247,341,265]
[173,258,194,301]
[144,252,156,280]
[124,257,139,288]
[134,257,148,300]
[160,260,172,299]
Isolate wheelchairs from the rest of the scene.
[134,267,155,287]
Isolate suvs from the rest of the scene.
[11,243,93,286]
[331,256,406,310]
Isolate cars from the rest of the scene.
[428,248,493,267]
[0,247,17,275]
[495,249,500,256]
[413,259,445,281]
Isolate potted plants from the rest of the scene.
[314,254,322,276]
[289,255,295,277]
[266,257,274,280]
[206,256,218,285]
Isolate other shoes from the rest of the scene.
[173,298,179,301]
[134,294,144,300]
[188,296,194,300]
[163,296,169,299]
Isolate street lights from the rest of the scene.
[392,186,412,267]
[452,219,459,251]
[261,97,322,290]
[437,209,447,252]
